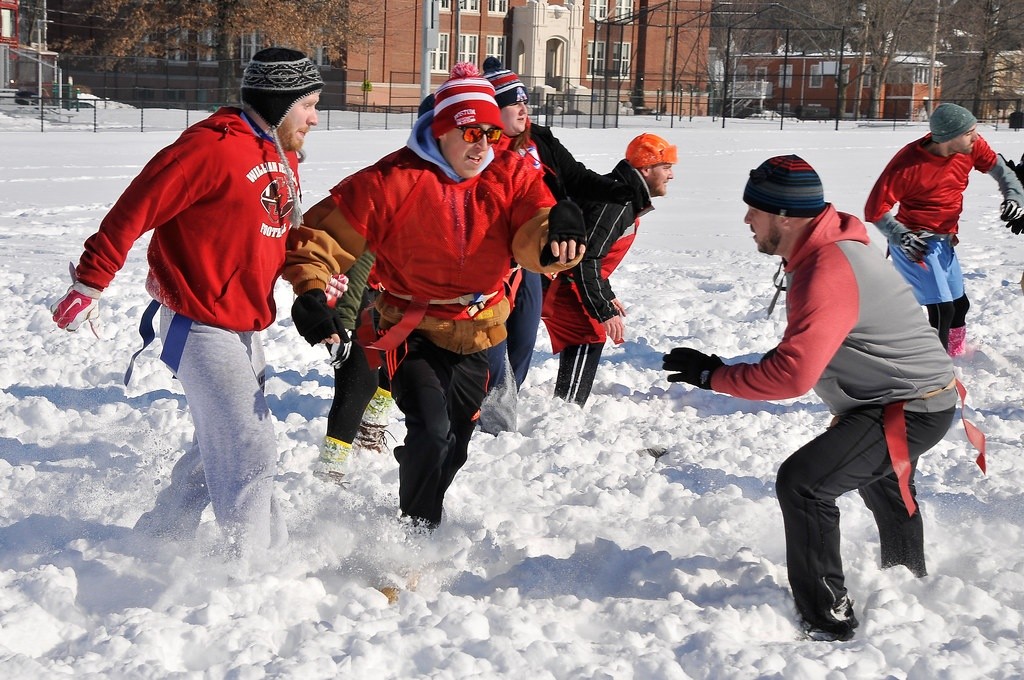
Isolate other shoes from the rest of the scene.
[352,420,396,454]
[800,613,855,642]
[313,471,350,489]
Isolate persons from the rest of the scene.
[540,133,678,409]
[49,48,349,548]
[283,61,586,535]
[313,93,436,481]
[481,57,637,394]
[662,154,987,642]
[864,104,1024,355]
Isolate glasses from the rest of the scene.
[455,126,503,144]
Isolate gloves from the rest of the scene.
[891,224,930,263]
[326,274,349,308]
[999,200,1024,235]
[661,347,724,390]
[325,329,354,370]
[49,281,102,332]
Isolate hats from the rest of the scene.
[418,93,436,119]
[930,102,976,143]
[626,133,677,168]
[241,48,325,228]
[742,155,826,217]
[481,57,529,107]
[432,63,506,140]
